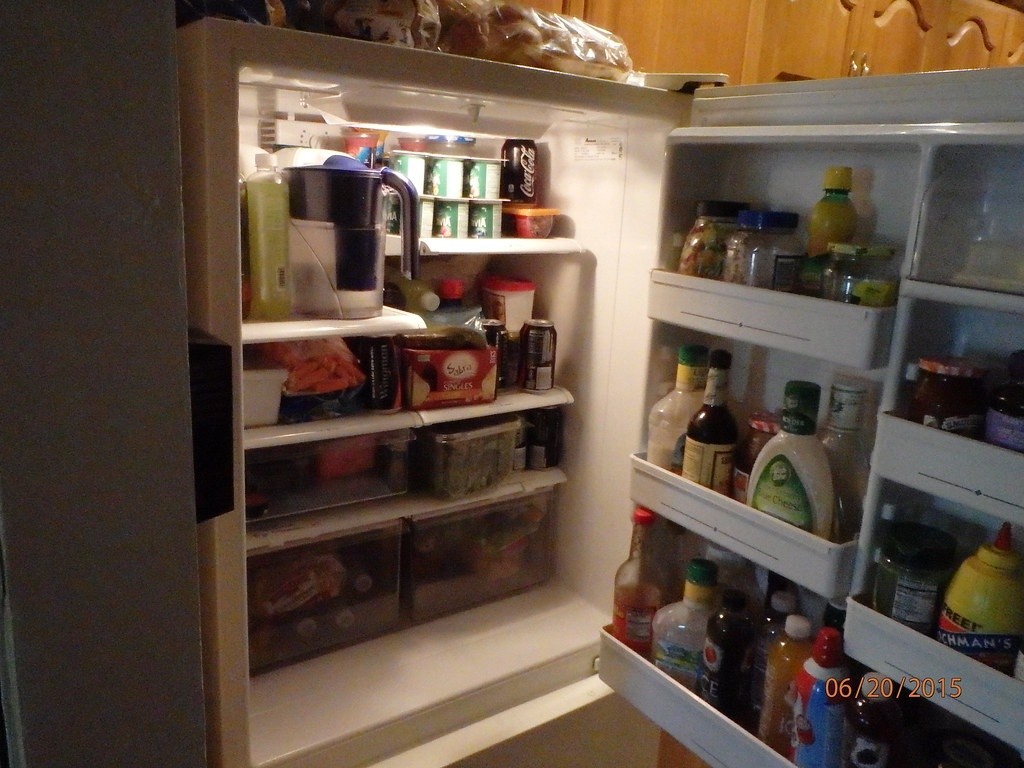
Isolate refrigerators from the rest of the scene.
[0,0,1024,768]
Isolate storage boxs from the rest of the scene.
[429,416,519,499]
[242,485,560,670]
[248,430,415,521]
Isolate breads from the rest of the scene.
[290,1,635,75]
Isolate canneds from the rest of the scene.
[481,318,509,390]
[674,198,904,311]
[729,414,781,503]
[903,355,986,440]
[871,523,958,639]
[915,728,1005,768]
[360,333,405,414]
[500,139,539,208]
[518,319,557,392]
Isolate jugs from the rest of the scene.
[274,146,422,322]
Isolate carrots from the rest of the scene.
[254,337,368,393]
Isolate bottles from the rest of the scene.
[935,520,1023,679]
[238,152,293,325]
[676,166,900,308]
[437,277,465,314]
[646,343,869,543]
[983,349,1024,455]
[383,265,441,314]
[609,505,904,768]
